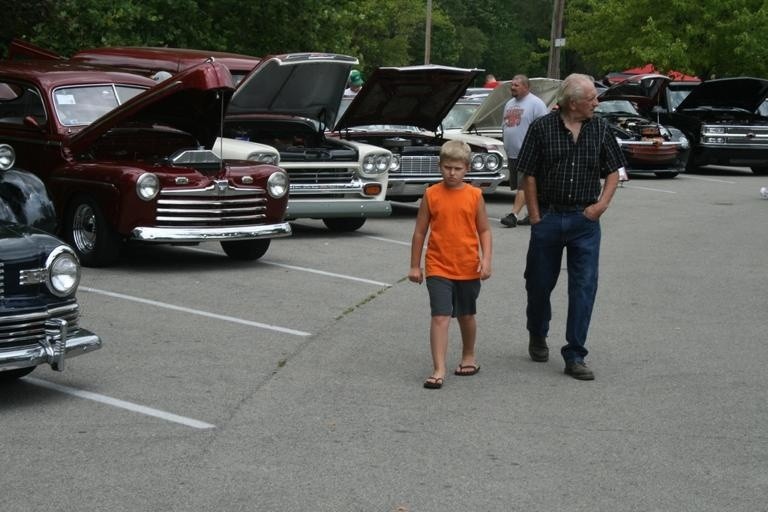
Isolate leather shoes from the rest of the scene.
[516,216,528,224]
[500,213,517,226]
[528,331,548,362]
[565,362,594,381]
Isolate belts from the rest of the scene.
[548,204,581,213]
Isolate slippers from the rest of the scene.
[423,375,443,389]
[454,359,480,376]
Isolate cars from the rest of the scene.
[1,227,103,376]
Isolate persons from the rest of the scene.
[407,140,492,389]
[500,74,549,228]
[514,73,628,381]
